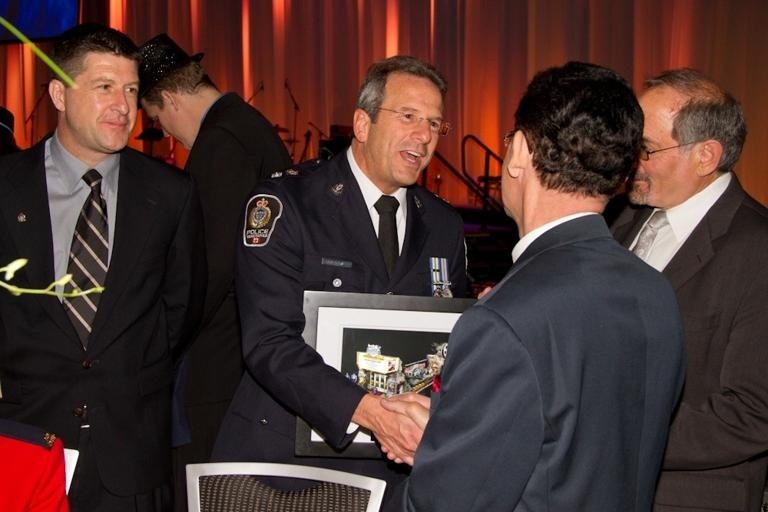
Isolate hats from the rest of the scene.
[137,33,204,110]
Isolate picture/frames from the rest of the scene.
[294,290,477,459]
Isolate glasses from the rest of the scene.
[504,129,533,155]
[366,105,451,137]
[642,139,706,160]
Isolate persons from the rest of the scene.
[1,27,208,510]
[135,32,296,510]
[605,67,767,510]
[208,55,466,511]
[379,59,685,511]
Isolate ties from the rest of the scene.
[373,196,400,275]
[632,210,671,261]
[61,169,109,352]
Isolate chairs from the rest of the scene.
[186,458,387,511]
[0,425,93,512]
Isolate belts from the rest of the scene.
[70,425,93,445]
